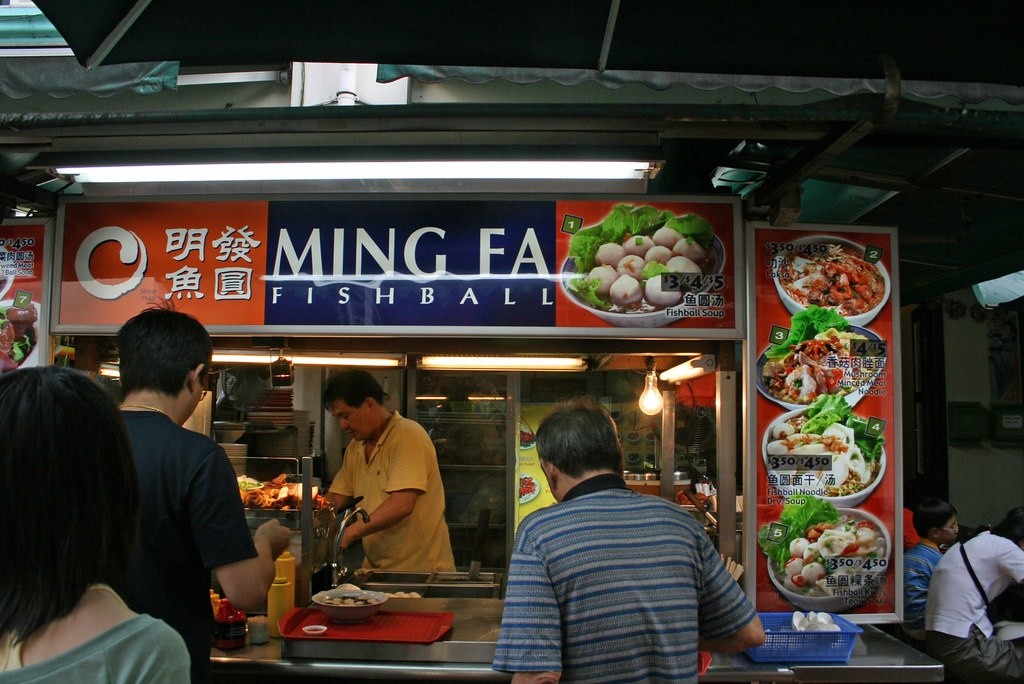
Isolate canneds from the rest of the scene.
[214,598,246,649]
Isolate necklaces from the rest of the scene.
[119,404,164,413]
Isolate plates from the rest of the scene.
[216,443,248,477]
[302,625,327,636]
[247,386,316,457]
[520,432,536,449]
[519,472,540,505]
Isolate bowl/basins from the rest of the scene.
[791,611,842,632]
[757,324,887,411]
[611,409,655,462]
[0,299,45,369]
[311,589,389,624]
[767,508,891,612]
[773,235,891,327]
[559,232,726,328]
[623,474,656,480]
[761,407,887,507]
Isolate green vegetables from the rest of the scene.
[239,480,259,491]
[0,306,31,361]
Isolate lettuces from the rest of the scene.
[800,393,885,461]
[764,305,848,358]
[568,203,712,310]
[757,495,840,569]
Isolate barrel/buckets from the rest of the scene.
[701,521,743,564]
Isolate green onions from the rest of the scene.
[804,521,879,592]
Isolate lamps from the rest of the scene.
[282,351,408,369]
[415,353,590,374]
[211,349,280,365]
[24,132,664,194]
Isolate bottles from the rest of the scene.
[208,587,246,649]
[275,549,296,607]
[266,569,292,638]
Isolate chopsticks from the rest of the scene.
[720,554,745,582]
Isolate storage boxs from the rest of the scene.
[280,590,505,663]
[745,609,863,664]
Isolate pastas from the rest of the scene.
[813,328,875,392]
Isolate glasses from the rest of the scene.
[943,520,959,531]
[196,366,207,401]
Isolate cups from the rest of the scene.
[247,623,270,644]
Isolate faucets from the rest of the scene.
[331,505,371,588]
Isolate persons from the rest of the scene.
[898,496,959,640]
[492,401,765,684]
[106,306,293,684]
[903,474,932,550]
[323,368,456,572]
[0,366,192,684]
[924,505,1024,684]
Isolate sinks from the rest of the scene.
[364,571,432,584]
[359,585,426,598]
[421,585,496,598]
[427,572,500,584]
